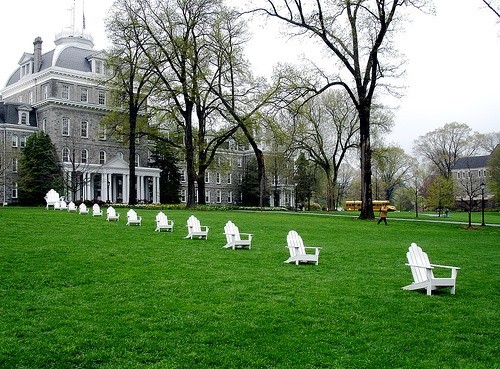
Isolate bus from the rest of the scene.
[344,200,397,212]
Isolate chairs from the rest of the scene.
[92,204,103,217]
[106,206,120,222]
[185,215,211,240]
[44,189,90,215]
[284,230,323,266]
[223,221,255,250]
[400,243,461,296]
[125,209,142,226]
[154,212,174,233]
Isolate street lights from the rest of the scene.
[480,182,487,226]
[294,181,299,212]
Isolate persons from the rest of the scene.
[377,203,389,226]
